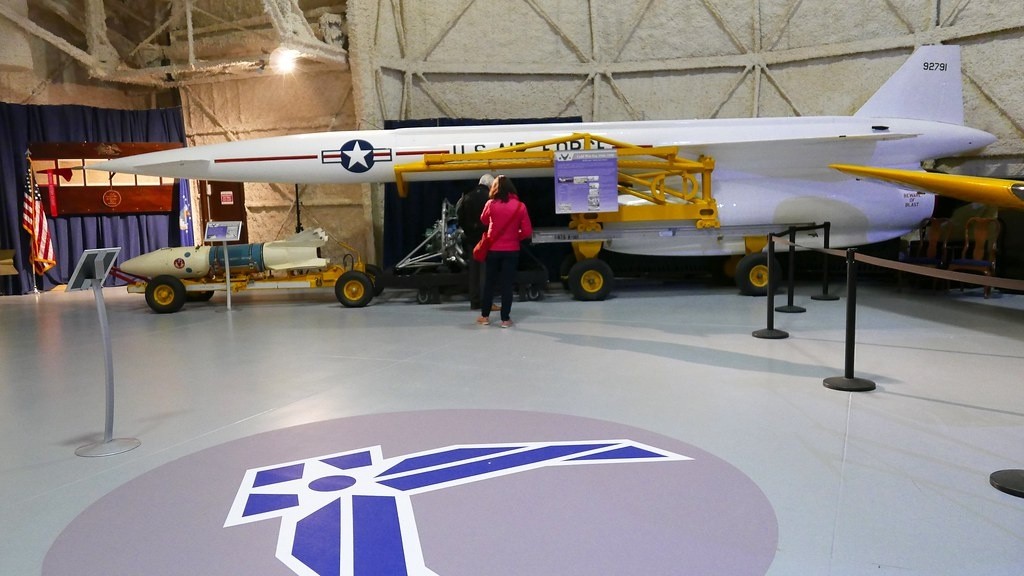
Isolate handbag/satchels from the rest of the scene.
[472,232,488,263]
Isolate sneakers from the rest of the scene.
[502,318,513,328]
[477,317,489,325]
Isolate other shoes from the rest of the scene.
[490,304,501,311]
[471,304,480,309]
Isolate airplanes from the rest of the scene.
[85,45,1024,301]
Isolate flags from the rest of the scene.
[179,177,194,247]
[22,157,56,275]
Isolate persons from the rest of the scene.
[477,174,533,327]
[454,174,502,310]
